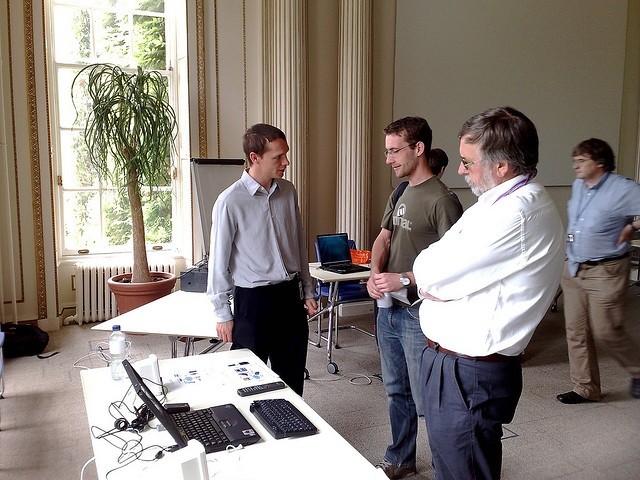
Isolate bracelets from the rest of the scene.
[630,222,640,232]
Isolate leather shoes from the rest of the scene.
[631,379,640,398]
[557,390,588,404]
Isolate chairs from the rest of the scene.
[307,232,377,350]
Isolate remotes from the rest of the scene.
[237,381,285,397]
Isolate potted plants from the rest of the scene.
[72,61,182,336]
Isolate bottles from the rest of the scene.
[108,325,129,380]
[376,291,394,309]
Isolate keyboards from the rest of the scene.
[250,399,317,439]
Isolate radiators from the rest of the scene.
[64,258,176,326]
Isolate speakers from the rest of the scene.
[170,439,210,480]
[133,354,165,396]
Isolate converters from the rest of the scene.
[164,403,190,414]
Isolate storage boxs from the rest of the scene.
[179,268,207,293]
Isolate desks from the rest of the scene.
[91,290,235,357]
[77,348,389,480]
[307,262,379,375]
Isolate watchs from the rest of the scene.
[399,271,411,288]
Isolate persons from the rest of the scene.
[207,124,318,398]
[429,147,448,179]
[556,138,640,400]
[413,104,565,480]
[367,116,463,480]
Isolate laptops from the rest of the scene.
[317,233,371,274]
[122,359,261,454]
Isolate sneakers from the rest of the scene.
[375,458,416,480]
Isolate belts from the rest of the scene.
[584,251,630,266]
[427,338,509,362]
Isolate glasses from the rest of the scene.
[384,142,416,156]
[461,157,484,169]
